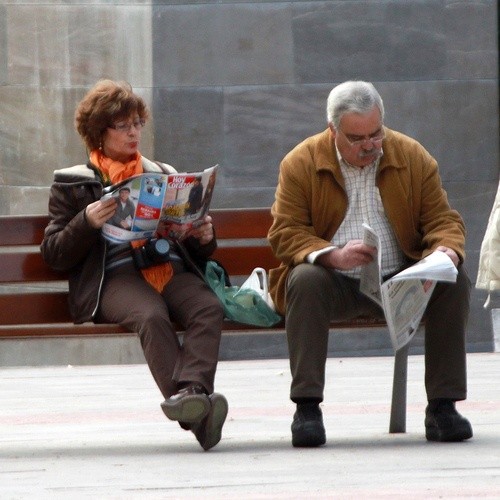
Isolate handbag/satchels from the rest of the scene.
[205,262,281,328]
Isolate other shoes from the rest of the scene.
[161,385,213,422]
[290,406,326,447]
[425,406,473,443]
[195,392,228,451]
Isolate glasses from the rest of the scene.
[109,116,142,132]
[334,125,387,147]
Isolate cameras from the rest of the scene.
[132,238,171,269]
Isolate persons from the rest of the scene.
[267,81,473,447]
[106,188,135,232]
[39,79,228,451]
[185,175,203,215]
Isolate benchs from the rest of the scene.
[0,207,425,432]
